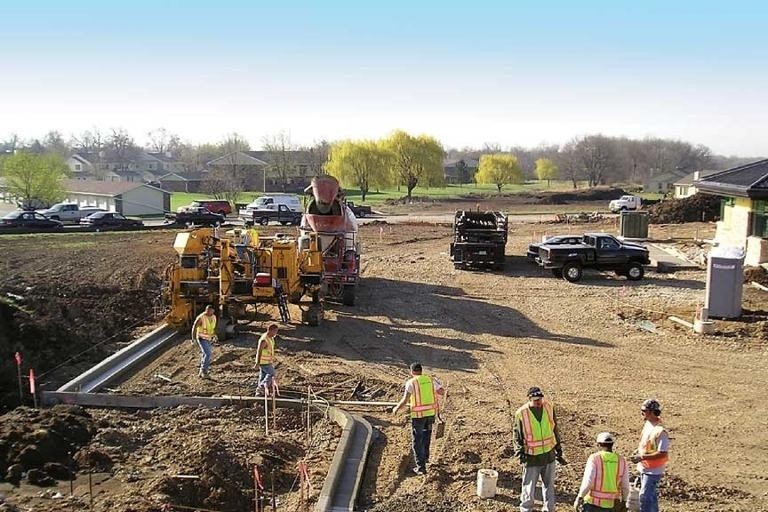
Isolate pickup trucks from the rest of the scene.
[34,203,108,224]
[535,235,651,283]
[165,206,224,227]
[345,202,371,217]
[238,203,303,226]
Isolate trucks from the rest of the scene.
[449,208,508,271]
[609,195,661,214]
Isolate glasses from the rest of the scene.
[641,409,656,413]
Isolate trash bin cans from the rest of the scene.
[620,211,649,238]
[706,250,746,318]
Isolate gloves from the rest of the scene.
[574,497,582,512]
[554,448,563,458]
[393,405,399,415]
[516,453,525,465]
[629,454,644,464]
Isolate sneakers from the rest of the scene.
[198,371,211,378]
[413,459,429,475]
[256,386,264,395]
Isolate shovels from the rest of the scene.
[436,416,445,439]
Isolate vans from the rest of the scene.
[245,196,300,212]
[178,200,232,218]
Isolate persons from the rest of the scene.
[514,387,562,511]
[394,363,442,474]
[627,400,669,511]
[574,431,630,512]
[191,305,216,379]
[255,323,278,396]
[237,222,258,267]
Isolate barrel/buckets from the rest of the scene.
[476,463,499,499]
[624,476,641,512]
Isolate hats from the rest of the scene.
[526,387,544,398]
[411,362,422,375]
[597,432,614,444]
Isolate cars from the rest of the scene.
[79,212,145,233]
[527,235,588,265]
[0,210,64,234]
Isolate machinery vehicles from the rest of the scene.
[298,176,360,307]
[168,227,325,340]
